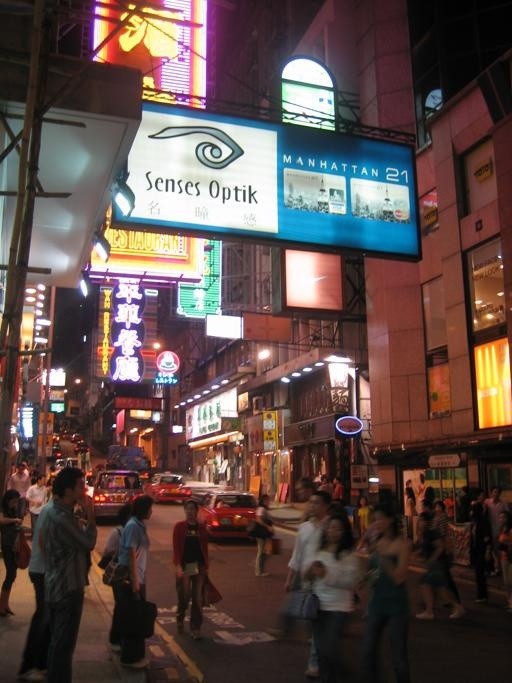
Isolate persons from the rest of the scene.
[285,475,512,683]
[104,503,132,653]
[117,494,154,669]
[172,501,209,641]
[255,494,273,577]
[0,458,98,683]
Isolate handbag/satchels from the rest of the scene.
[175,561,200,577]
[98,554,119,586]
[247,519,271,537]
[115,597,156,636]
[281,590,319,620]
[13,530,31,568]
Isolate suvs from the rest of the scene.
[90,468,148,519]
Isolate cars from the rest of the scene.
[49,429,153,497]
[191,490,259,542]
[144,469,193,505]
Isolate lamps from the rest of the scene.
[91,228,111,262]
[80,266,94,299]
[110,163,136,218]
